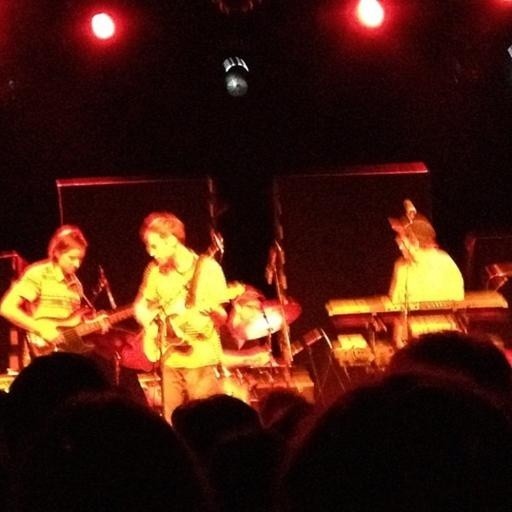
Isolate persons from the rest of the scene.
[1,225,88,366]
[133,213,231,425]
[390,233,412,348]
[402,215,464,338]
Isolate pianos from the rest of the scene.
[323,291,510,332]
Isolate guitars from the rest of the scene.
[25,303,135,358]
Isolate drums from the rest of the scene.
[237,366,314,402]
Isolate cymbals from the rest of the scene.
[228,301,302,338]
